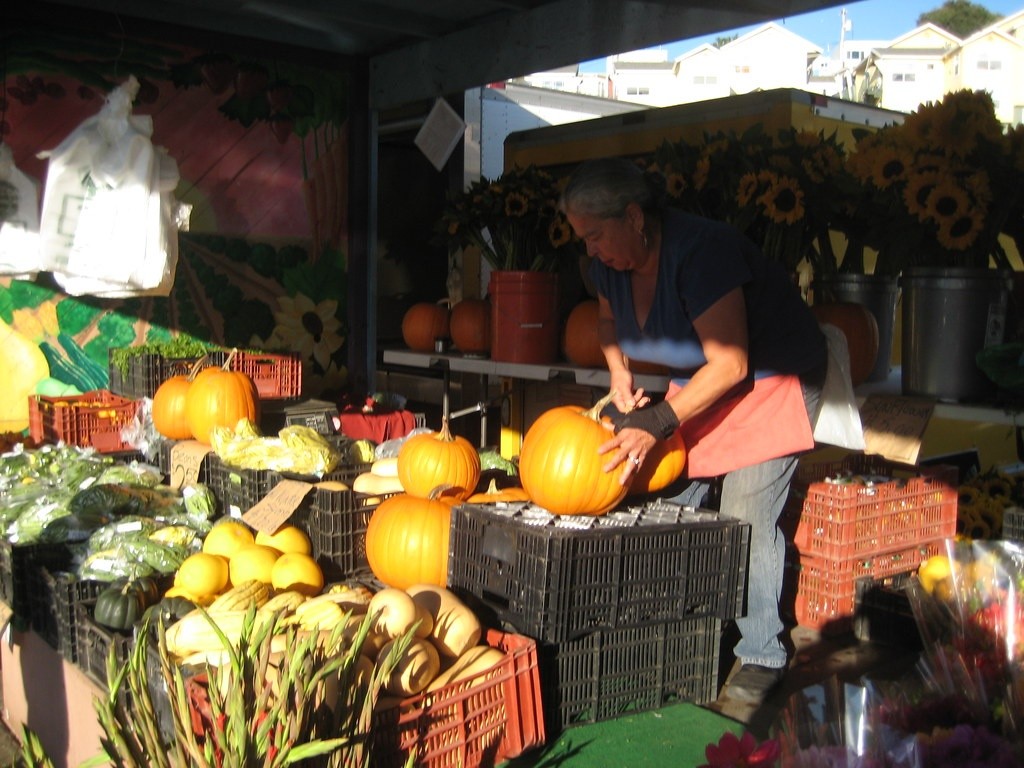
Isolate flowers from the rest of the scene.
[695,540,1024,768]
[441,90,1024,270]
[942,459,1024,541]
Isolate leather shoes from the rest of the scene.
[726,664,782,706]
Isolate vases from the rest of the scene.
[488,270,562,365]
[899,268,1014,400]
[824,274,898,380]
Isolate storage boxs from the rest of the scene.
[0,344,959,768]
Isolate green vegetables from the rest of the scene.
[0,444,219,586]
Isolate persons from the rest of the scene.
[559,160,829,704]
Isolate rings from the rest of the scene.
[628,456,639,465]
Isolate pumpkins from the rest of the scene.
[94,295,879,719]
